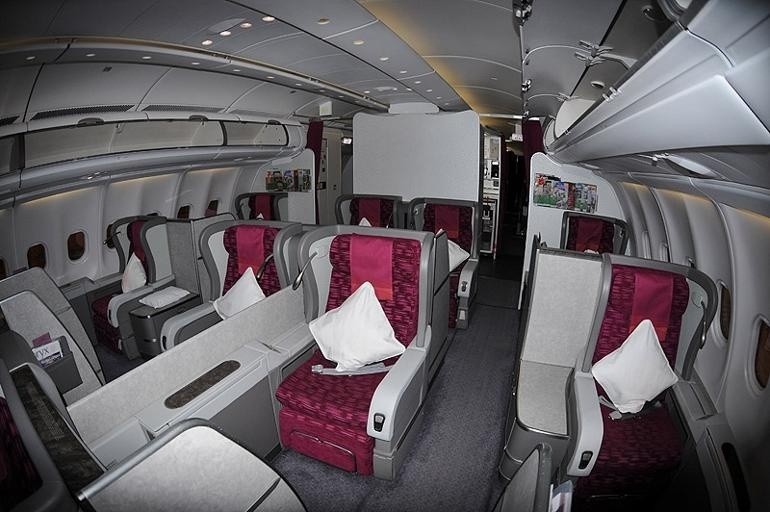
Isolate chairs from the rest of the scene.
[0,191,719,512]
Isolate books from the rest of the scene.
[30,338,64,369]
[31,331,52,348]
[547,482,554,512]
[553,479,574,512]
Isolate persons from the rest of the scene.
[543,183,548,194]
[265,169,311,193]
